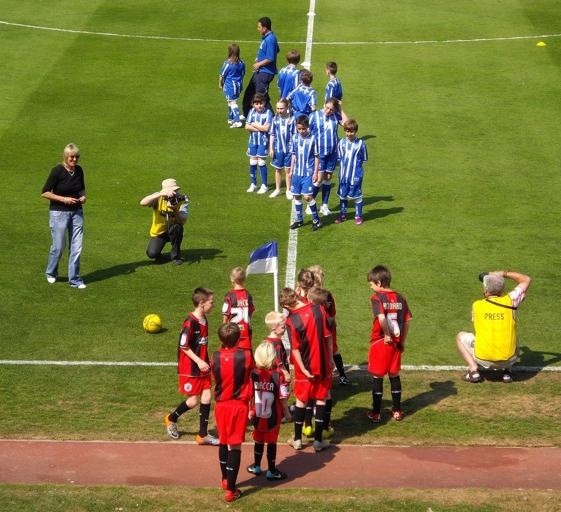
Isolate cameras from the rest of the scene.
[170,194,186,206]
[478,272,489,283]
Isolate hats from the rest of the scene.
[160,178,180,191]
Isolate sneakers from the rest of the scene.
[221,480,241,502]
[367,409,402,423]
[228,115,246,129]
[47,274,56,283]
[501,370,512,382]
[247,182,293,200]
[196,434,219,445]
[289,204,362,231]
[165,414,179,439]
[78,283,86,288]
[247,464,287,480]
[288,426,334,451]
[463,369,481,383]
[338,376,350,384]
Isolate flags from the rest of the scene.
[245,239,279,279]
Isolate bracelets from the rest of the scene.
[173,212,182,218]
[63,197,68,203]
[503,271,506,278]
[505,271,508,277]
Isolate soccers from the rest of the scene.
[143,314,161,332]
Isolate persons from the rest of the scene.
[217,16,370,232]
[453,266,532,385]
[361,264,413,425]
[162,267,257,501]
[40,142,88,290]
[247,264,350,479]
[139,176,190,267]
